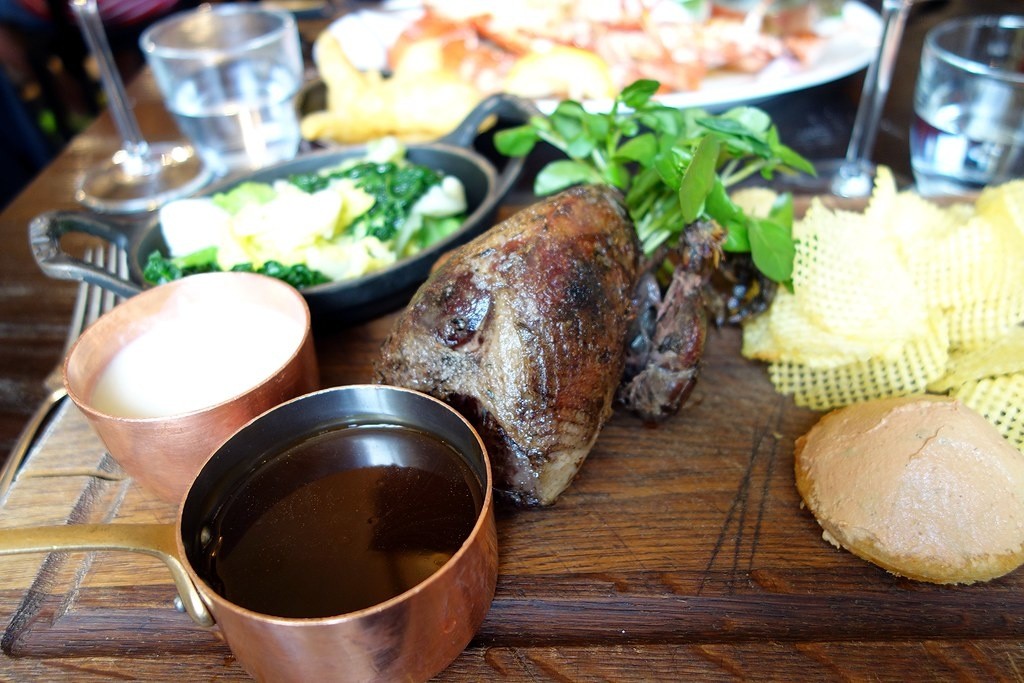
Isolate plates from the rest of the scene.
[314,0,885,114]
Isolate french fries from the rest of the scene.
[299,30,495,148]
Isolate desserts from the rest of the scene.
[794,395,1024,584]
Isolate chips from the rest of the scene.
[717,164,1024,455]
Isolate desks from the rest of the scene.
[1,1,324,483]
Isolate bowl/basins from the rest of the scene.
[0,384,496,683]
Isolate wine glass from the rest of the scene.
[74,0,216,213]
[787,0,916,209]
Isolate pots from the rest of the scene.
[29,92,538,329]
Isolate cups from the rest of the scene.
[62,276,319,501]
[140,6,303,174]
[910,19,1024,198]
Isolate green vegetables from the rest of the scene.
[141,156,464,289]
[494,82,817,291]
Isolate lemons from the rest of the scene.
[503,46,617,101]
